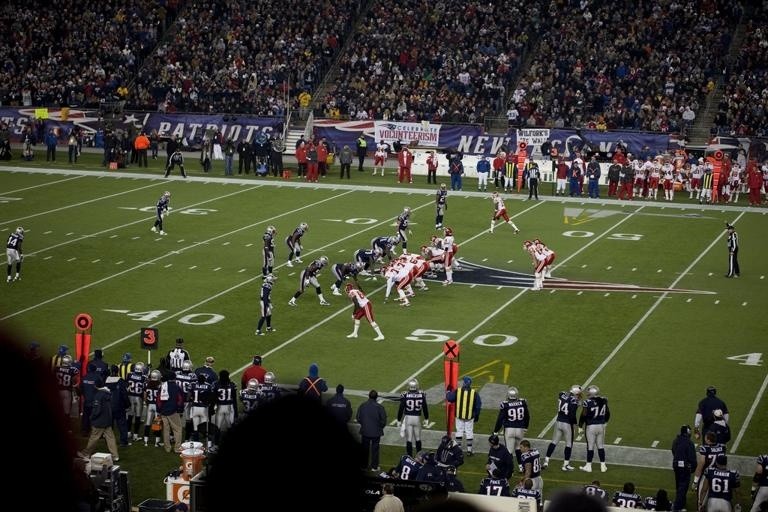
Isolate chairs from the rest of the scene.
[374,335,384,341]
[466,450,607,473]
[253,326,278,337]
[347,333,358,338]
[531,273,551,290]
[329,283,344,296]
[393,280,452,307]
[286,255,304,268]
[151,227,168,236]
[287,299,332,307]
[112,432,182,461]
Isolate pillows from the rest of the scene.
[681,426,691,438]
[522,239,541,250]
[320,255,330,265]
[354,205,453,277]
[54,344,276,390]
[163,191,171,199]
[266,274,277,284]
[707,387,716,396]
[300,223,308,231]
[15,227,23,235]
[267,225,275,234]
[408,379,600,400]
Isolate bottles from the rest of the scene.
[713,408,723,418]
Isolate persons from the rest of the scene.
[721,216,744,281]
[1,338,768,511]
[4,226,28,284]
[251,184,556,345]
[0,1,764,207]
[150,189,172,239]
